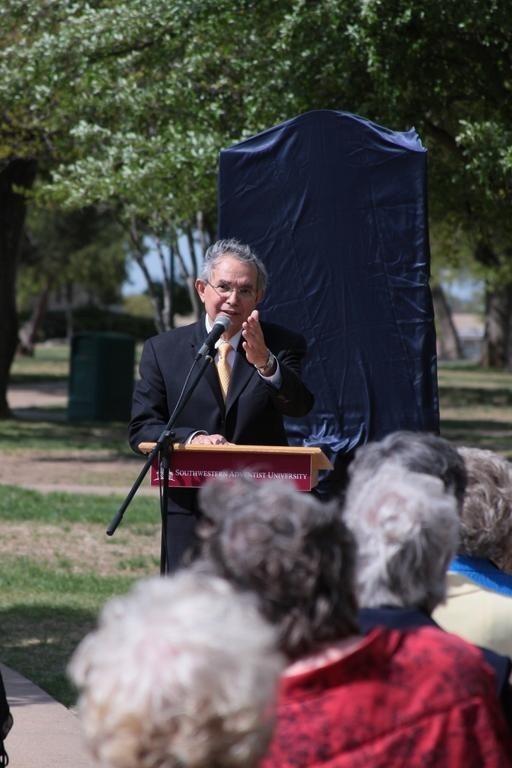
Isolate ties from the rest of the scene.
[215,340,233,402]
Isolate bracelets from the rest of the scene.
[255,349,275,374]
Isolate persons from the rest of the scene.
[446,443,510,593]
[346,428,509,662]
[128,236,317,577]
[62,560,287,766]
[194,469,512,767]
[339,468,510,725]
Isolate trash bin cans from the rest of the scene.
[66,332,134,423]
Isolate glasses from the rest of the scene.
[206,279,256,299]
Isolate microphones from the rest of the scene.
[195,315,231,361]
